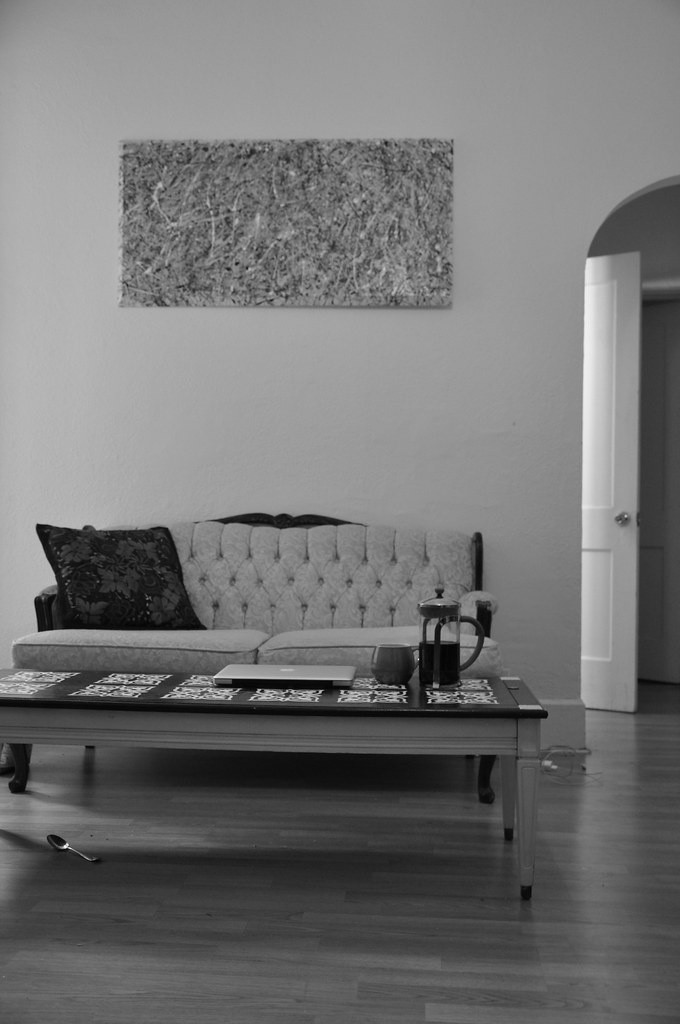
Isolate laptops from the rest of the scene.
[212,664,356,689]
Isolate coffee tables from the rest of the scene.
[0,666,551,904]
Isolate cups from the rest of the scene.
[371,643,420,686]
[417,587,485,690]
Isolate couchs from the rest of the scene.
[8,512,505,808]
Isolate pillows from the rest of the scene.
[35,522,211,634]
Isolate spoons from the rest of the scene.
[46,833,102,862]
